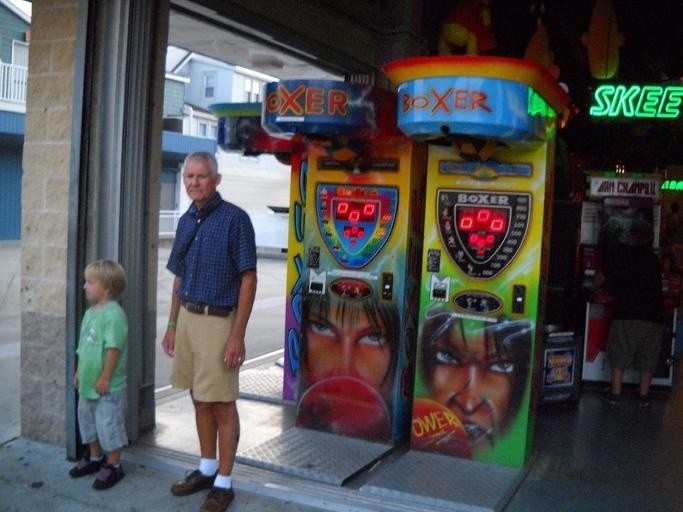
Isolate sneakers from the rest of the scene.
[601,386,650,408]
[69,453,106,476]
[93,463,125,491]
[201,482,234,512]
[171,468,219,496]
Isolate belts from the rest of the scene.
[179,297,233,318]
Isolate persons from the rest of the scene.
[592,220,665,409]
[419,310,532,452]
[69,260,130,490]
[160,150,257,512]
[299,273,400,420]
[668,202,683,239]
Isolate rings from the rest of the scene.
[238,357,241,361]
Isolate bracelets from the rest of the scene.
[168,322,176,327]
